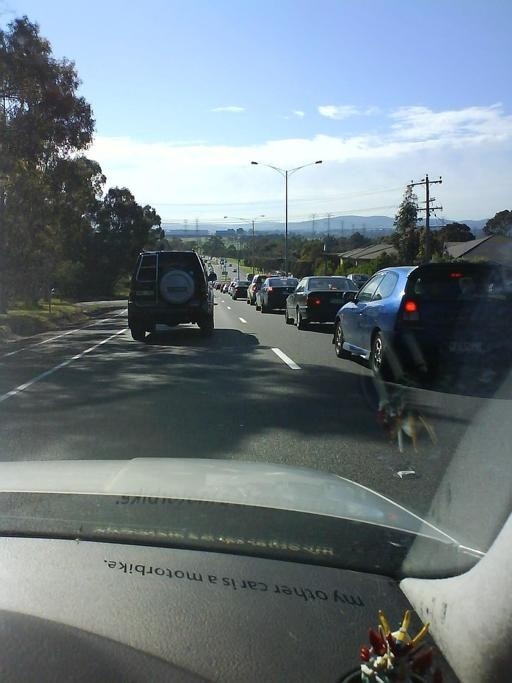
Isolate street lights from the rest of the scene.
[224,215,264,273]
[251,161,323,277]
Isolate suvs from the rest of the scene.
[128,249,218,340]
